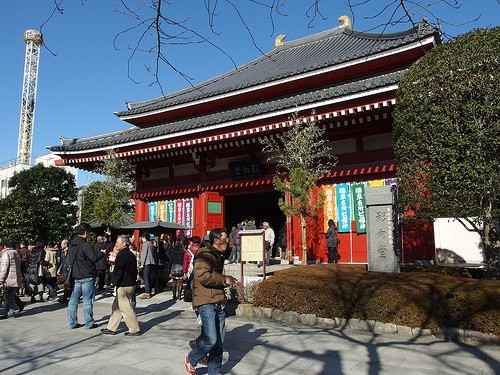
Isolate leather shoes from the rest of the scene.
[124,331,142,336]
[101,328,116,335]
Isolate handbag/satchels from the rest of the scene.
[265,241,270,251]
[64,265,72,288]
[38,264,45,276]
[172,263,184,277]
[0,282,8,302]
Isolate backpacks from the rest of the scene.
[186,249,194,280]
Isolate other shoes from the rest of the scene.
[39,299,45,302]
[17,294,24,297]
[31,298,37,303]
[90,324,98,329]
[74,324,81,329]
[48,296,59,303]
[57,296,67,304]
[189,341,207,365]
[139,293,150,299]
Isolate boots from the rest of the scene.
[172,286,178,300]
[177,287,182,300]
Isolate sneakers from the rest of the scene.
[185,354,196,375]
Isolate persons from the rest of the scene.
[68,223,106,328]
[227,217,287,265]
[184,228,238,375]
[93,231,209,302]
[101,235,142,336]
[326,219,338,264]
[0,239,75,319]
